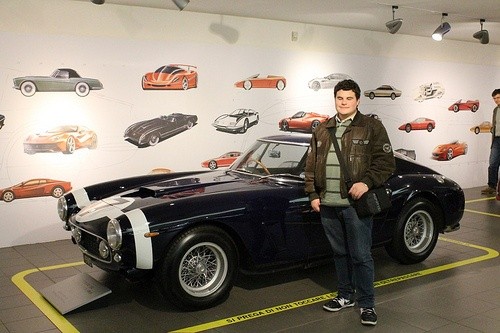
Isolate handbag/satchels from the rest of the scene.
[345,177,394,217]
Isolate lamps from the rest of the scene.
[431,12,451,41]
[472,19,489,44]
[173,0,190,10]
[384,5,402,35]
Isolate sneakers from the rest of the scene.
[359,307,378,325]
[322,295,356,312]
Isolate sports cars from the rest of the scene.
[58,135,465,311]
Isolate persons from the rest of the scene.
[481,89,500,199]
[304,78,396,325]
